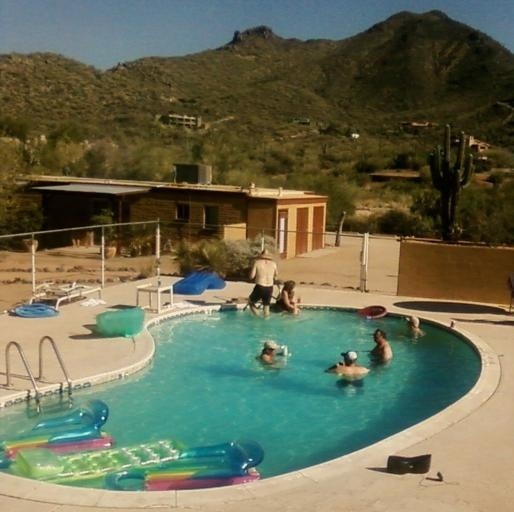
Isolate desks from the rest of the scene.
[137,285,174,313]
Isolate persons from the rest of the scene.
[325,350,371,396]
[84,226,94,247]
[275,280,301,315]
[369,328,394,367]
[259,340,284,370]
[397,315,427,343]
[70,230,80,247]
[247,248,279,319]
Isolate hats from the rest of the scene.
[260,249,271,259]
[406,316,419,326]
[341,351,357,361]
[265,341,277,349]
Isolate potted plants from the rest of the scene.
[22,202,49,251]
[105,240,117,259]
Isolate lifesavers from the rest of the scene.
[104,468,149,492]
